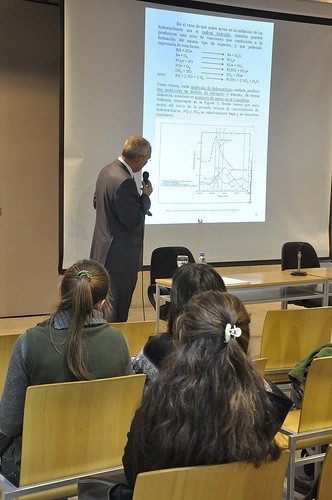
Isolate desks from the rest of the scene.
[154,269,326,335]
[282,267,332,309]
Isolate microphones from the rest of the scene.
[143,172,149,182]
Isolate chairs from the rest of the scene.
[0,241,332,500]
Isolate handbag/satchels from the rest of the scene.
[78,472,133,500]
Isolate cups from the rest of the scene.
[176,255,189,268]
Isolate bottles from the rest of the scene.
[199,253,207,265]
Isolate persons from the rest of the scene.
[0,258,294,500]
[88,134,153,324]
